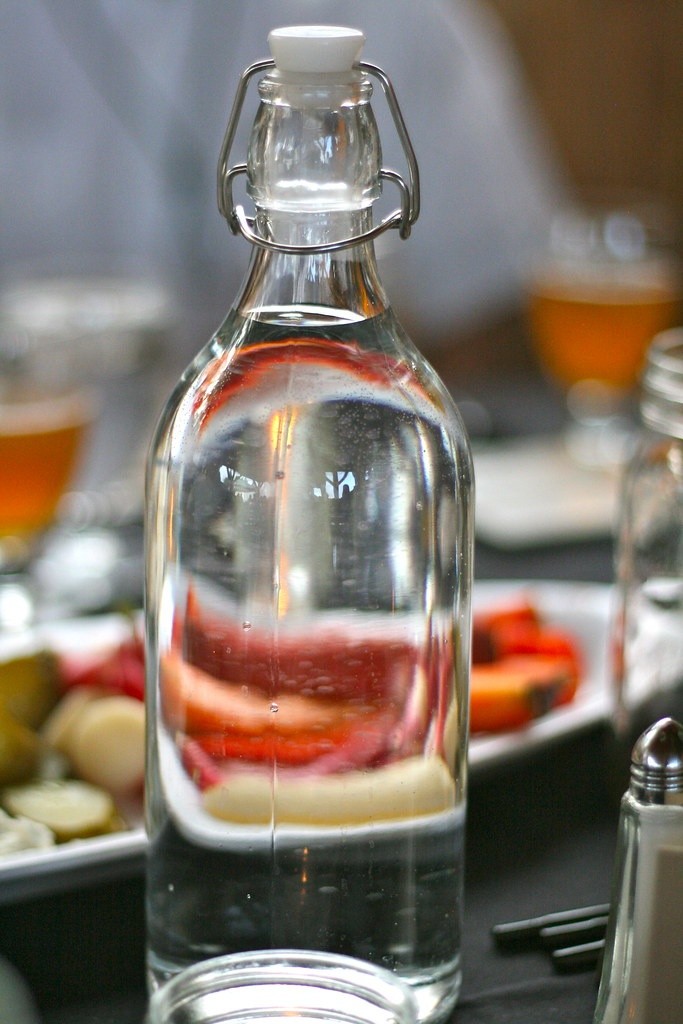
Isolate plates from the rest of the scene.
[0,573,683,912]
[448,441,621,553]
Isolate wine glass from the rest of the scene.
[526,212,678,509]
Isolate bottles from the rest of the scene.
[598,330,682,822]
[135,27,480,1023]
[593,715,682,1023]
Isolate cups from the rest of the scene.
[1,327,94,575]
